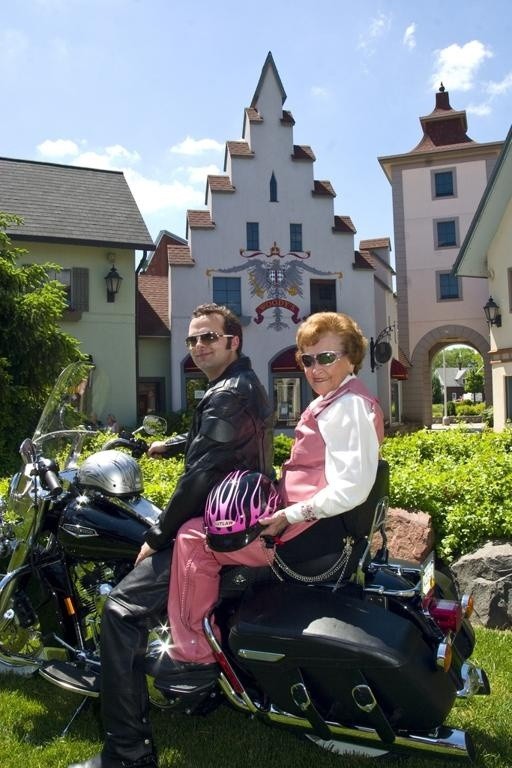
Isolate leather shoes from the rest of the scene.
[67,747,159,768]
[142,650,222,679]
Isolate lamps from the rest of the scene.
[483,295,501,328]
[104,264,123,303]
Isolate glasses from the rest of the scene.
[299,349,348,368]
[184,331,235,348]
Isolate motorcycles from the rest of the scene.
[1,361,490,764]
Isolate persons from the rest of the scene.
[139,310,385,676]
[62,300,277,766]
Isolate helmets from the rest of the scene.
[73,448,146,497]
[202,468,284,553]
